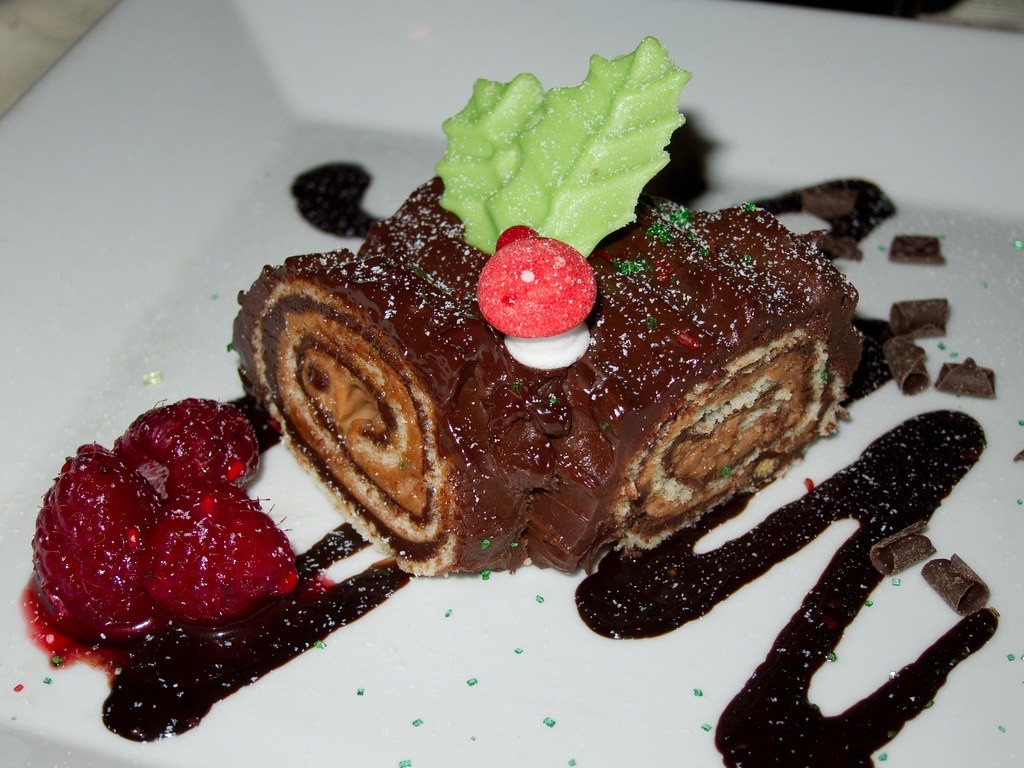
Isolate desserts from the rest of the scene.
[227,36,865,576]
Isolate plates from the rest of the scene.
[0,0,1024,768]
